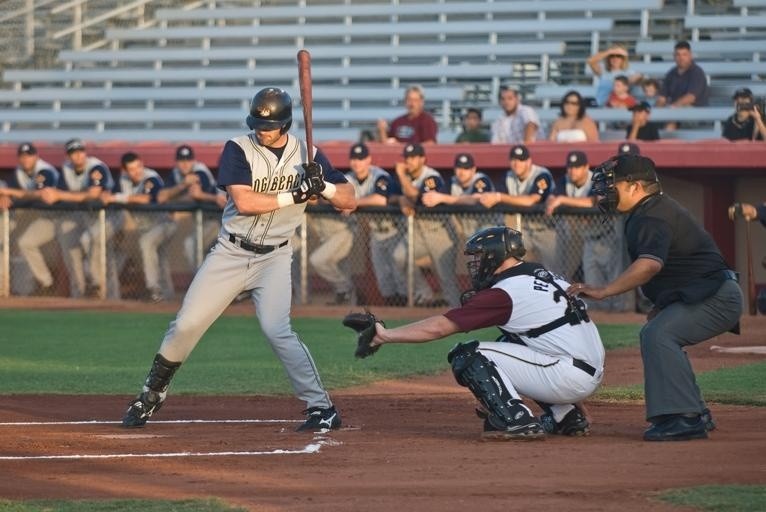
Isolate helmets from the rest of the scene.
[463,226,526,291]
[591,155,656,225]
[246,87,292,134]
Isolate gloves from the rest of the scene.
[292,162,326,204]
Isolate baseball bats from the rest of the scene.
[298,51,319,200]
[746,219,757,315]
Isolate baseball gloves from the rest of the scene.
[343,311,384,359]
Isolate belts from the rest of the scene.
[573,358,595,377]
[723,270,737,280]
[229,234,288,254]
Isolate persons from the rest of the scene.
[99,145,226,304]
[550,92,599,141]
[457,108,491,142]
[376,86,437,145]
[490,86,542,143]
[589,46,642,106]
[725,195,766,318]
[565,154,746,436]
[0,140,115,299]
[642,41,708,130]
[545,141,643,306]
[719,87,766,144]
[120,85,359,430]
[307,142,557,306]
[369,223,606,441]
[627,101,661,139]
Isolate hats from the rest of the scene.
[348,145,368,160]
[731,87,752,99]
[566,151,586,167]
[176,145,193,160]
[401,143,424,157]
[66,139,84,153]
[454,154,474,169]
[618,143,639,154]
[628,100,650,112]
[17,143,36,154]
[510,146,528,161]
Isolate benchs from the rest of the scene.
[1,1,766,143]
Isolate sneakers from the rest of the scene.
[298,405,341,429]
[483,418,545,440]
[123,385,167,426]
[643,408,715,442]
[540,407,592,436]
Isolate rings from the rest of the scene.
[578,282,582,288]
